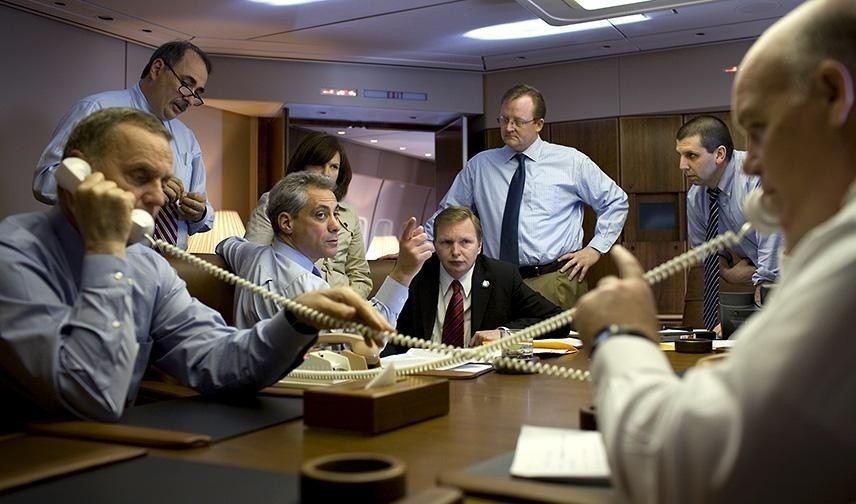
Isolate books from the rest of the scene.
[510,421,613,485]
[380,332,584,383]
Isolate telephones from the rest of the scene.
[744,186,781,236]
[307,332,382,371]
[54,157,155,244]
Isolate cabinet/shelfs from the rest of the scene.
[486,110,748,191]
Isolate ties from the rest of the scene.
[499,152,526,271]
[151,197,178,254]
[441,281,465,354]
[702,188,723,333]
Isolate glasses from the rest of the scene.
[163,62,207,107]
[496,114,538,128]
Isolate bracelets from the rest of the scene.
[587,321,657,356]
[498,322,513,342]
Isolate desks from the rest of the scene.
[0,324,747,504]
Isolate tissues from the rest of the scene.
[302,360,450,435]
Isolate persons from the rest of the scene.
[572,0,854,503]
[0,105,393,427]
[245,126,377,306]
[380,200,572,358]
[29,36,216,263]
[419,82,632,334]
[677,114,786,326]
[213,168,437,368]
